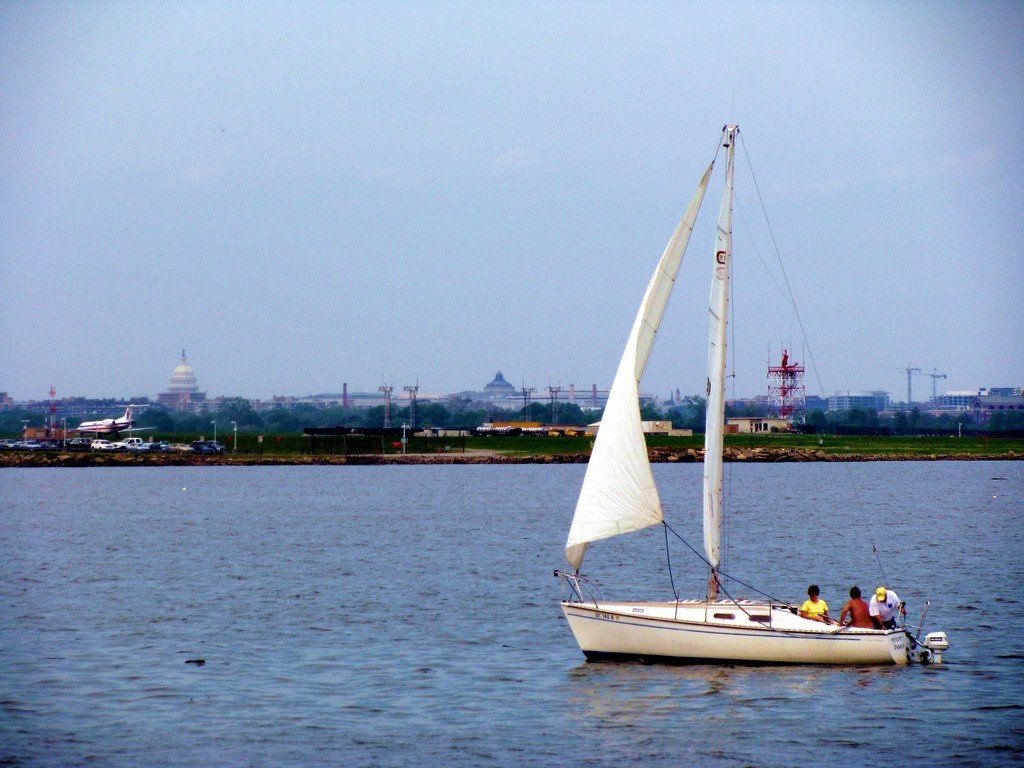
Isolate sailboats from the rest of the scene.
[551,119,953,667]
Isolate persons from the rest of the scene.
[869,587,906,630]
[801,585,835,624]
[837,586,874,628]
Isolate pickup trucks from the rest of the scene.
[116,438,155,453]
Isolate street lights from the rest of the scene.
[61,418,67,447]
[231,421,237,449]
[21,420,30,441]
[211,421,217,441]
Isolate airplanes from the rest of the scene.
[76,404,151,438]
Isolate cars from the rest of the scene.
[1,439,41,449]
[91,440,115,452]
[190,441,215,455]
[70,438,92,448]
[208,440,226,453]
[41,441,58,447]
[168,443,194,454]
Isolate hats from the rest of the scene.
[876,587,886,601]
